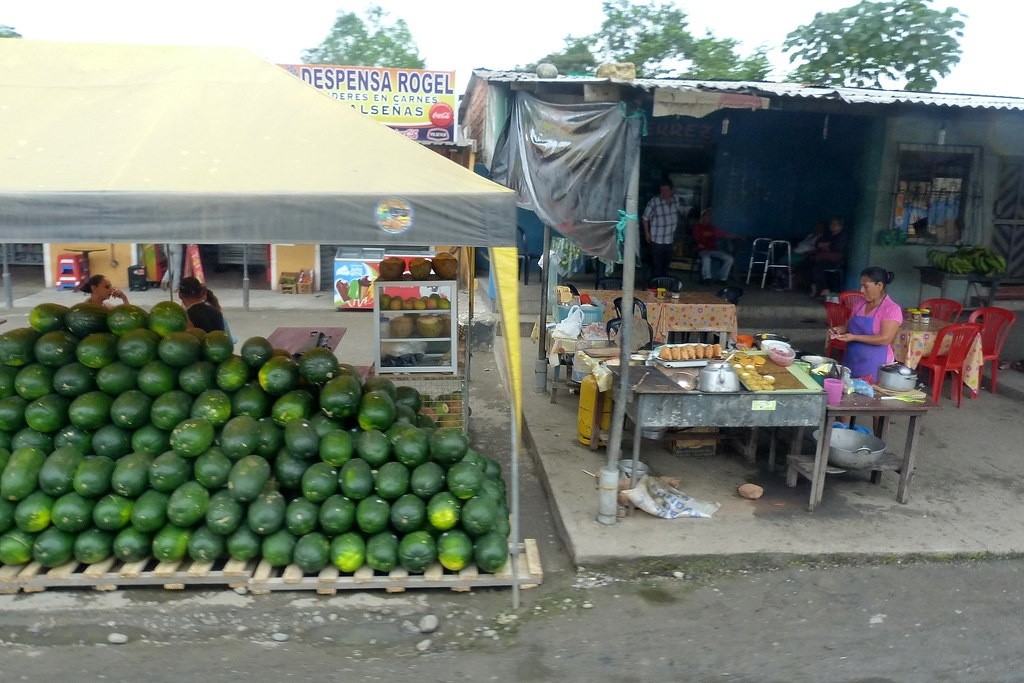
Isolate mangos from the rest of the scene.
[380,293,450,310]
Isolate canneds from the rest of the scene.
[906,308,931,324]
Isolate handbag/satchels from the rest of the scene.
[551,304,585,340]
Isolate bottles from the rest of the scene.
[907,308,930,324]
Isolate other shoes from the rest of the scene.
[767,282,776,288]
[809,290,822,298]
[717,279,731,285]
[776,286,788,292]
[704,279,712,287]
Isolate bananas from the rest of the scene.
[928,244,1007,276]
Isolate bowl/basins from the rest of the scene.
[753,332,796,366]
[801,354,851,376]
[668,372,699,391]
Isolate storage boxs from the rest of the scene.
[552,295,605,325]
[279,270,315,294]
[667,426,719,454]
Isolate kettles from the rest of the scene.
[698,346,740,393]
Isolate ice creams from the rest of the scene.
[358,275,372,305]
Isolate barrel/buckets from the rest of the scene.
[616,459,648,491]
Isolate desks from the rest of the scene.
[63,248,107,280]
[267,327,347,355]
[578,289,738,350]
[914,265,1004,311]
[891,318,985,395]
[531,322,617,404]
[365,334,470,437]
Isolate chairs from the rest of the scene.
[516,225,542,286]
[824,291,1017,409]
[563,275,744,352]
[688,240,723,283]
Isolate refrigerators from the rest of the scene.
[334,247,451,312]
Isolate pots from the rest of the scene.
[812,420,887,471]
[877,361,918,392]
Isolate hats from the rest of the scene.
[701,207,712,217]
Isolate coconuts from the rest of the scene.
[389,316,414,338]
[378,252,459,280]
[416,315,443,338]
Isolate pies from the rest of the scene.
[660,343,722,360]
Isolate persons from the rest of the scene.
[178,277,230,334]
[829,268,903,424]
[80,275,128,306]
[693,209,745,286]
[771,217,852,299]
[642,179,678,276]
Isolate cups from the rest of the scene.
[824,378,844,405]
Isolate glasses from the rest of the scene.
[104,285,110,289]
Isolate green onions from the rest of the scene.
[880,389,925,402]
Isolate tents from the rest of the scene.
[0,38,522,609]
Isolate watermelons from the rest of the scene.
[0,300,511,574]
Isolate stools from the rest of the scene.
[55,252,90,293]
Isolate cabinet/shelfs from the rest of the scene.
[0,243,43,265]
[788,378,945,512]
[373,274,458,375]
